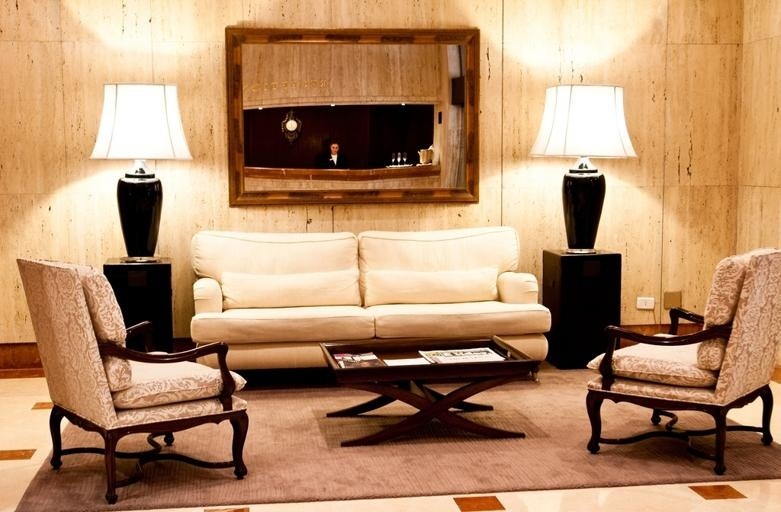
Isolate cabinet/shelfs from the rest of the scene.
[543,248,621,369]
[102,256,172,353]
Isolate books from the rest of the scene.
[333,351,386,368]
[418,346,505,363]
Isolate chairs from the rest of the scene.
[15,257,250,504]
[586,246,781,476]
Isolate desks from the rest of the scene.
[320,335,541,447]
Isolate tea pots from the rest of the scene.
[417,149,433,164]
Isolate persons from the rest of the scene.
[317,143,346,169]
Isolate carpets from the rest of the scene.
[14,360,781,511]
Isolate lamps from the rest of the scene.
[89,83,195,260]
[526,85,638,255]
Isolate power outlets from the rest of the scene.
[636,297,656,311]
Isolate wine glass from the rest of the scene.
[392,152,407,165]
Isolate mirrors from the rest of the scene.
[225,26,481,207]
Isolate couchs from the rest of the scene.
[192,225,556,389]
